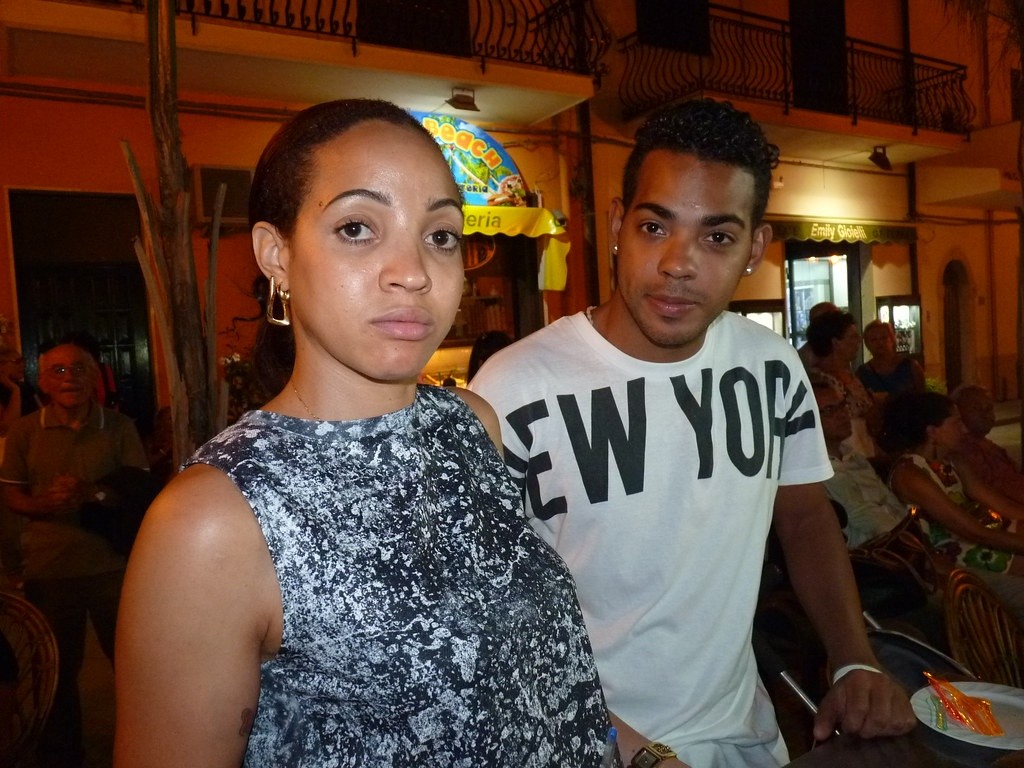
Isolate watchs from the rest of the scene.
[627,742,678,768]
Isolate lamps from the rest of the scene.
[868,145,894,171]
[445,86,480,112]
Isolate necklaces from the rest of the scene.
[289,375,322,421]
[587,305,596,325]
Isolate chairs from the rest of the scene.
[945,569,1024,690]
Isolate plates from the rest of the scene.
[909,681,1024,752]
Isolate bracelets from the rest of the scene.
[832,664,883,684]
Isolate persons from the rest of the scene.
[117,96,622,768]
[0,345,160,768]
[468,94,1024,768]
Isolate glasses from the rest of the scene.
[841,332,860,345]
[820,398,848,416]
[43,361,89,380]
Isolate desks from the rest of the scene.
[782,674,1024,768]
